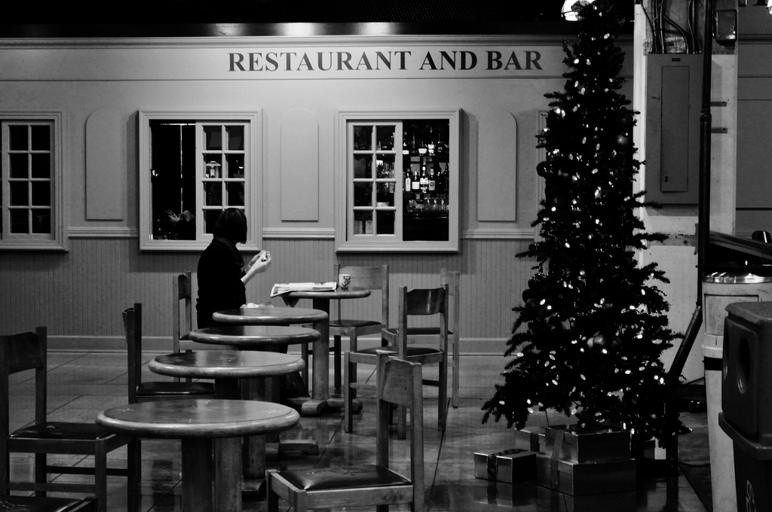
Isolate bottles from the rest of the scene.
[405,166,449,195]
[403,128,446,156]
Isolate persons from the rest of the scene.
[194,206,290,396]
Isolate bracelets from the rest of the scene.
[248,260,254,265]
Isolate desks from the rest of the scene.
[272,283,371,417]
[148,349,306,481]
[212,305,328,326]
[189,324,321,354]
[96,399,300,512]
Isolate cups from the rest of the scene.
[339,274,351,290]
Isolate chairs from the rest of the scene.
[1,325,143,512]
[173,271,240,352]
[264,348,426,511]
[381,268,460,409]
[121,302,215,404]
[344,283,449,433]
[300,264,389,398]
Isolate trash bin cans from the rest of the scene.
[700,271,772,512]
